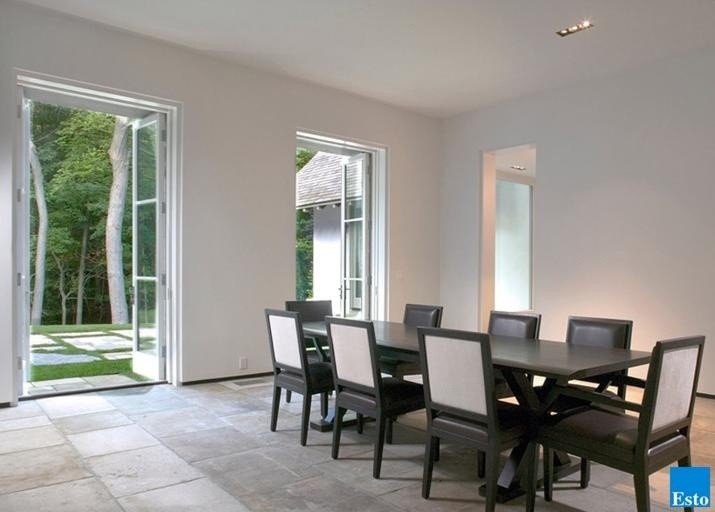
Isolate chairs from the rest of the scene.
[264,300,705,512]
